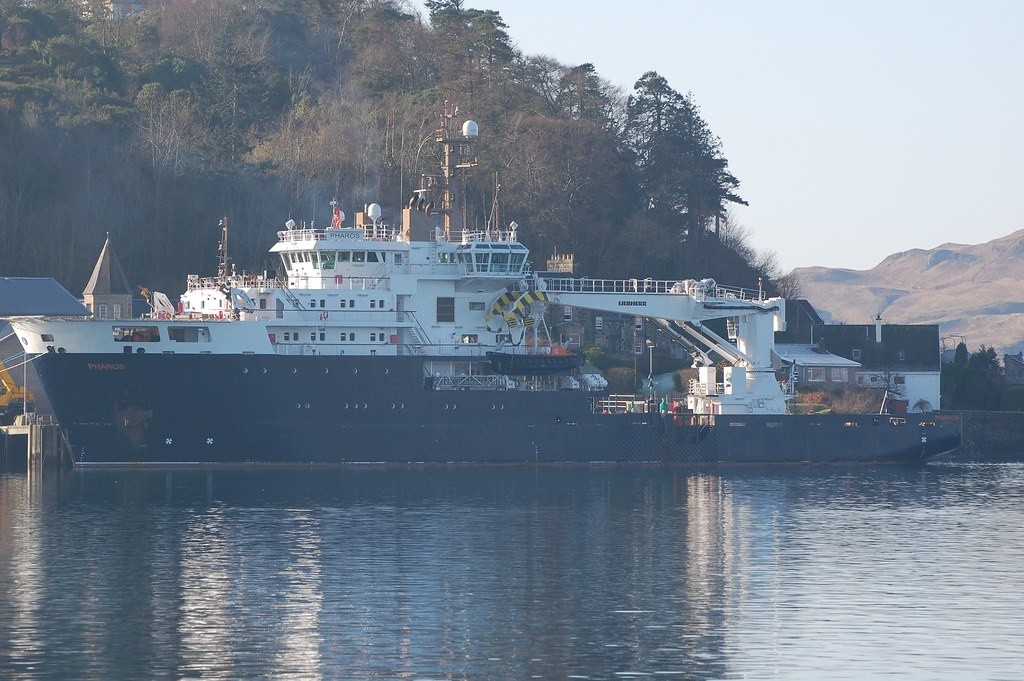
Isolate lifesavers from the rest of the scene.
[323,310,329,319]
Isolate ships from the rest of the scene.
[0,120,964,474]
